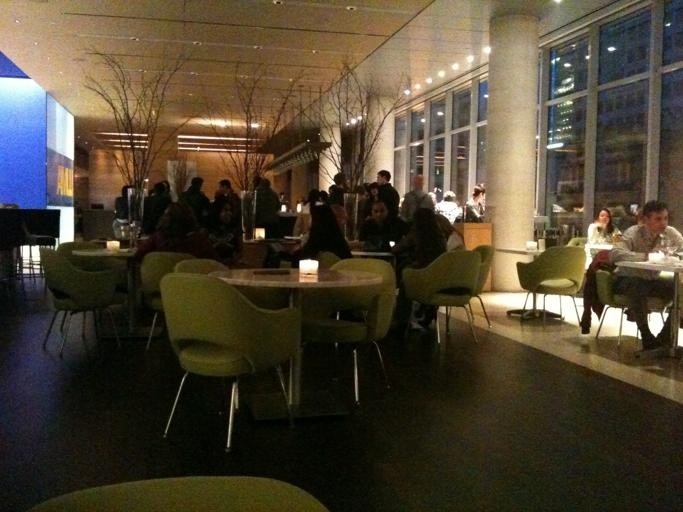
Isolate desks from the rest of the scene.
[214,271,383,403]
[618,255,683,352]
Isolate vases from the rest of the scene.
[124,184,145,243]
[342,189,362,244]
[239,190,256,242]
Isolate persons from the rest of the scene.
[369,182,379,199]
[391,207,448,325]
[143,181,173,235]
[116,186,133,218]
[250,177,282,237]
[610,200,682,352]
[330,174,346,203]
[427,191,438,205]
[270,202,352,264]
[414,213,465,327]
[401,175,433,215]
[359,202,405,243]
[434,191,462,223]
[375,170,401,211]
[204,198,243,258]
[218,180,240,218]
[136,199,209,264]
[309,190,329,203]
[212,190,228,227]
[177,178,208,205]
[465,184,486,220]
[584,209,622,269]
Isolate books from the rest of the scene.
[281,234,303,245]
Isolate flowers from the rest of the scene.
[201,59,311,240]
[77,41,208,223]
[289,62,409,241]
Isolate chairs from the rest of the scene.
[41,248,122,359]
[20,221,57,283]
[138,251,189,351]
[447,243,500,330]
[305,258,397,405]
[404,247,482,344]
[515,246,585,325]
[316,250,338,265]
[174,256,236,273]
[567,237,594,248]
[159,274,305,451]
[593,267,673,354]
[29,476,331,512]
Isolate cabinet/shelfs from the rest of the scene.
[448,216,496,292]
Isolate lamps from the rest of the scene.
[301,258,319,272]
[650,251,664,262]
[254,228,266,240]
[105,240,121,250]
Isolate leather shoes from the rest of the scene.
[656,334,670,343]
[642,339,664,349]
